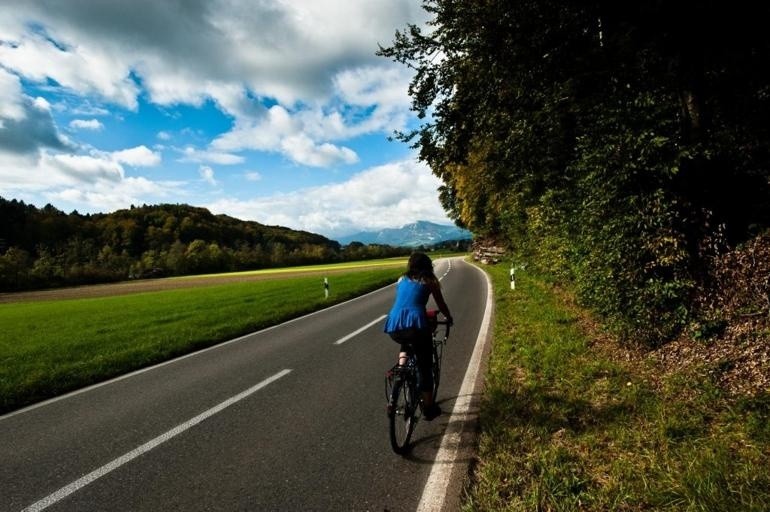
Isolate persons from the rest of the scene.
[381,252,454,421]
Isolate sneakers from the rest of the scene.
[423,402,441,417]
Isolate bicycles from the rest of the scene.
[385,319,453,455]
